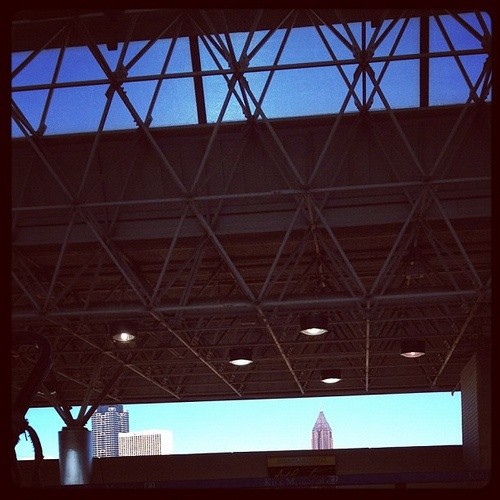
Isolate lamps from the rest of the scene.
[111,318,137,343]
[299,315,330,336]
[320,367,342,384]
[229,348,253,366]
[400,341,427,358]
